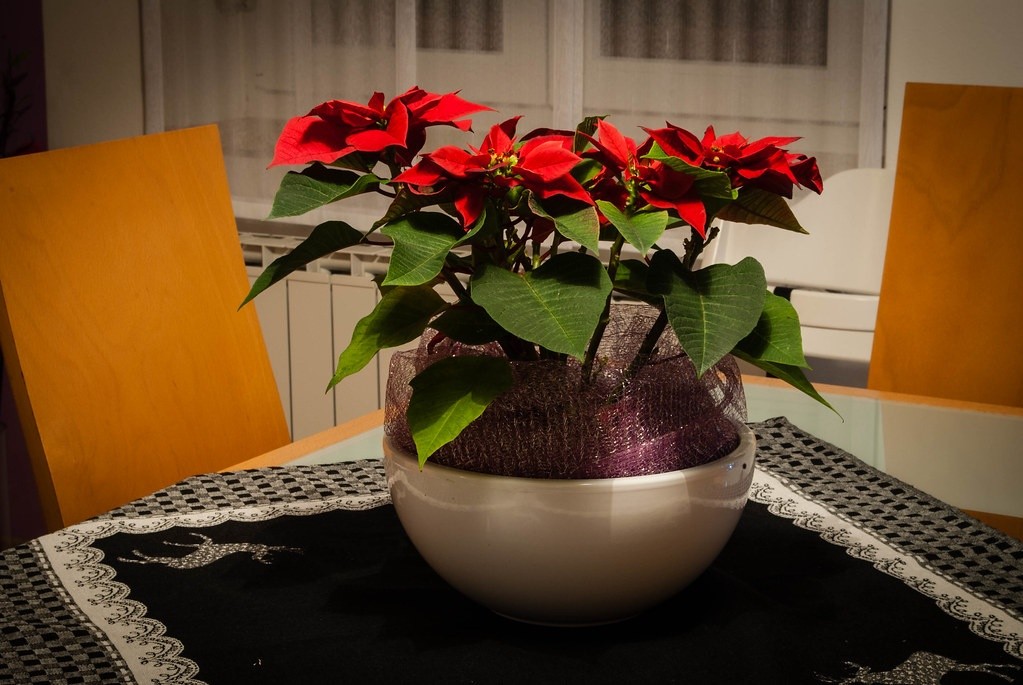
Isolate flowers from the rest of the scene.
[264,85,844,473]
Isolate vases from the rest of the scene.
[387,413,756,620]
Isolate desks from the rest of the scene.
[0,409,1023,685]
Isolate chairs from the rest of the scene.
[867,82,1023,409]
[0,125,291,524]
